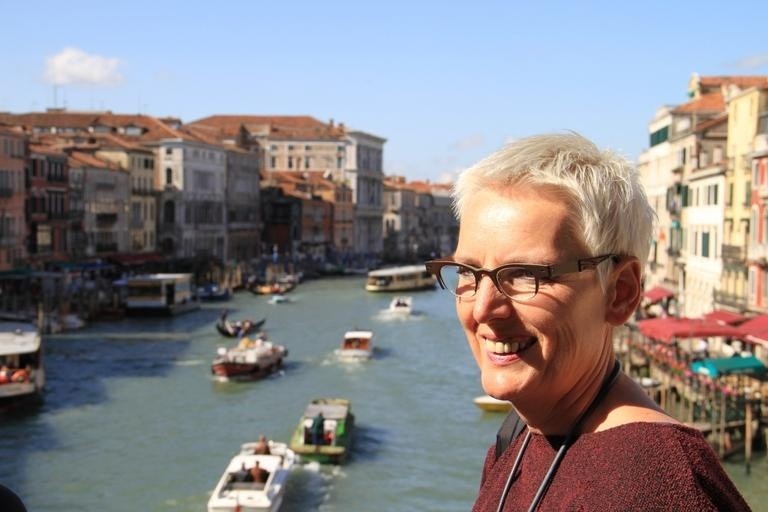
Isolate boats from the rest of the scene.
[204,438,299,512]
[391,295,414,316]
[0,307,88,336]
[120,271,200,317]
[215,317,269,337]
[363,264,438,293]
[206,333,285,382]
[287,397,358,463]
[334,328,376,361]
[472,394,512,414]
[0,319,48,414]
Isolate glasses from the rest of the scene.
[425,253,618,301]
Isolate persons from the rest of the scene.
[0,364,13,384]
[309,411,328,445]
[421,124,751,512]
[10,365,34,383]
[251,434,271,455]
[248,461,269,485]
[230,462,249,485]
